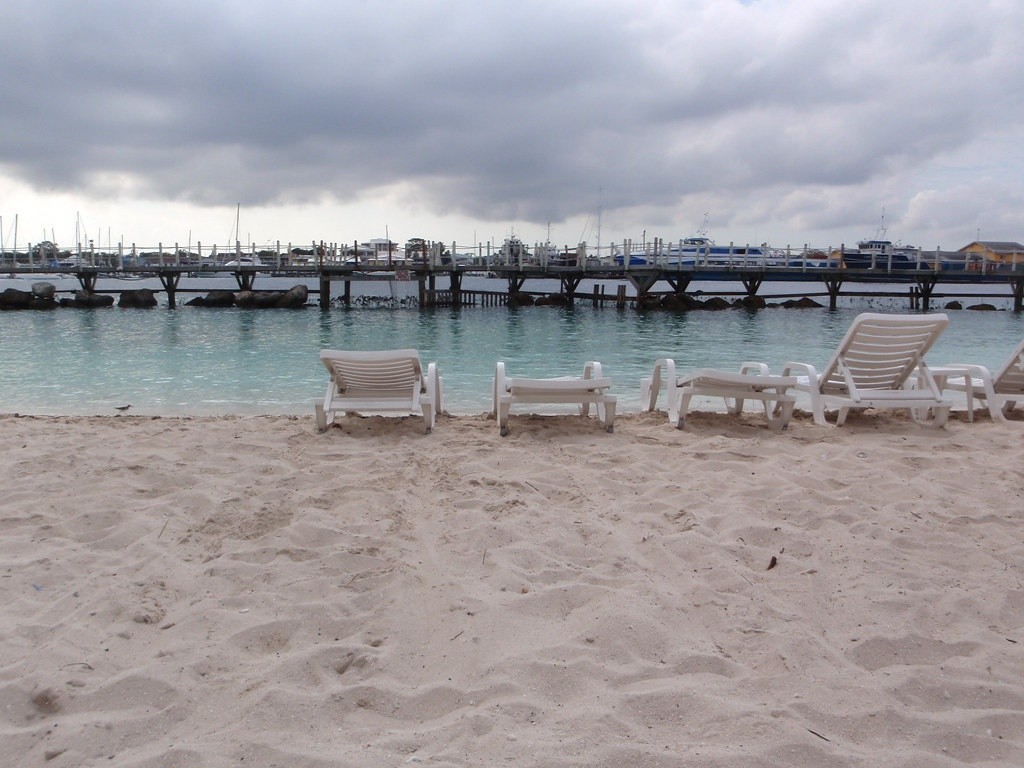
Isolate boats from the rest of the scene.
[616,243,841,282]
[843,240,928,270]
[0,202,611,280]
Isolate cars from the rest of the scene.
[224,256,259,266]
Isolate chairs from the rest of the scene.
[640,357,799,430]
[761,312,949,429]
[314,348,441,432]
[492,360,618,436]
[902,339,1024,427]
